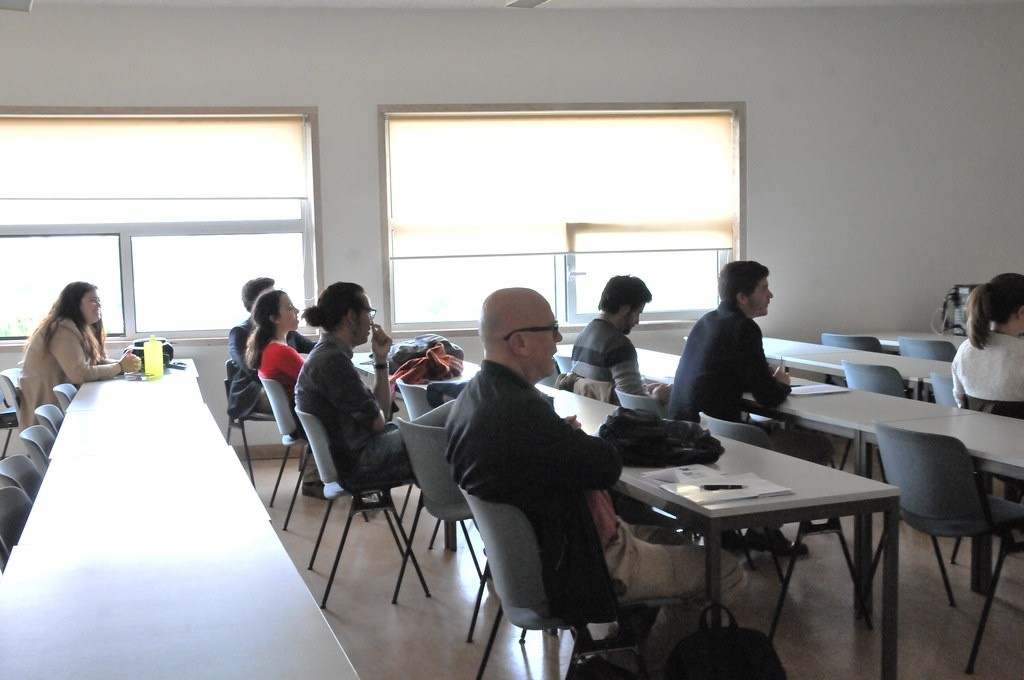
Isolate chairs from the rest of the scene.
[839,361,908,398]
[699,411,875,648]
[898,336,955,361]
[871,421,1024,675]
[820,334,884,353]
[0,348,667,680]
[928,372,957,408]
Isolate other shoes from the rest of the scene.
[302,481,326,499]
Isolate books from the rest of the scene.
[661,471,795,505]
[789,384,849,395]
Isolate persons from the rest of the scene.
[445,288,748,680]
[16,281,142,432]
[951,273,1024,553]
[227,277,326,499]
[571,276,671,406]
[669,261,809,556]
[245,290,305,399]
[293,282,416,492]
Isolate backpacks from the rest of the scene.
[667,605,787,680]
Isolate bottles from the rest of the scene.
[143,335,165,380]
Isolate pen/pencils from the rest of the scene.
[789,384,803,387]
[701,484,750,491]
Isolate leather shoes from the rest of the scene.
[720,530,745,548]
[745,528,808,555]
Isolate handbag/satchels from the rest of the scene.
[599,407,725,466]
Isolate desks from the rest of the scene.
[96,357,200,377]
[352,333,1024,680]
[52,401,228,459]
[64,377,204,413]
[0,545,360,680]
[18,460,272,546]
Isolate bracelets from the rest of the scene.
[119,363,123,373]
[373,361,387,368]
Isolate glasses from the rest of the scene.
[355,306,377,319]
[504,323,558,340]
